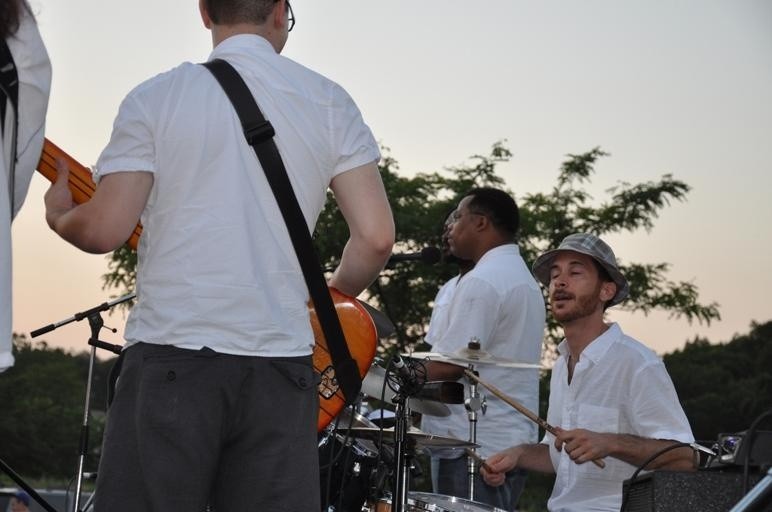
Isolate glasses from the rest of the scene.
[284,1,295,32]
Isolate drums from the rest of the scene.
[361,490,512,512]
[317,407,394,512]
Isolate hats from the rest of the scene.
[532,232,629,306]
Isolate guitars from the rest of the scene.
[35,137,378,434]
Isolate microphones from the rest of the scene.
[389,247,442,262]
[388,356,426,395]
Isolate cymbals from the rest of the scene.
[333,423,482,450]
[400,349,547,371]
[359,362,451,417]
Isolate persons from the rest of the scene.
[404,186,546,511]
[475,230,702,511]
[0,0,56,379]
[41,0,396,506]
